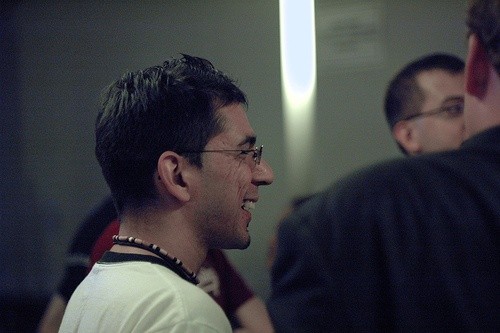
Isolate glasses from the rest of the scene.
[177,145,264,165]
[405,104,464,120]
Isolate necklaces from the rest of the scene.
[112,234,201,285]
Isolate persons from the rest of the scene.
[57,55,275,333]
[383,51,465,156]
[272,0,500,333]
[37,0,500,333]
